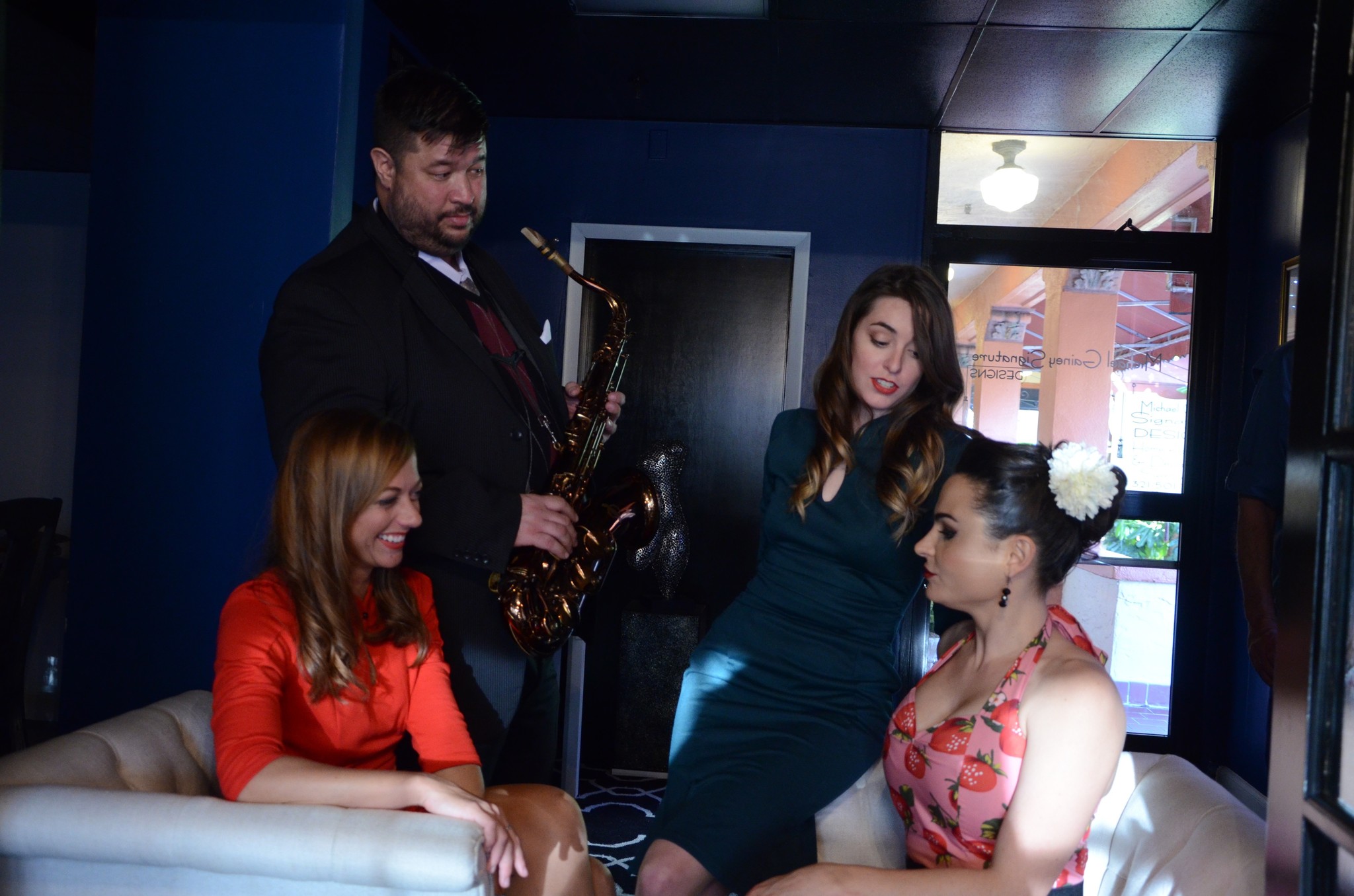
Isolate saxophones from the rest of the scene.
[483,221,667,662]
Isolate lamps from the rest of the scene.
[980,140,1039,212]
[955,204,976,226]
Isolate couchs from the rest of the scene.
[1082,752,1267,896]
[0,690,496,896]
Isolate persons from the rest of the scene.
[744,440,1127,896]
[635,267,991,896]
[210,412,615,895]
[264,70,625,789]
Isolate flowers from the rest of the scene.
[1047,440,1118,522]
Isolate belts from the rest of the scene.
[904,857,1085,896]
